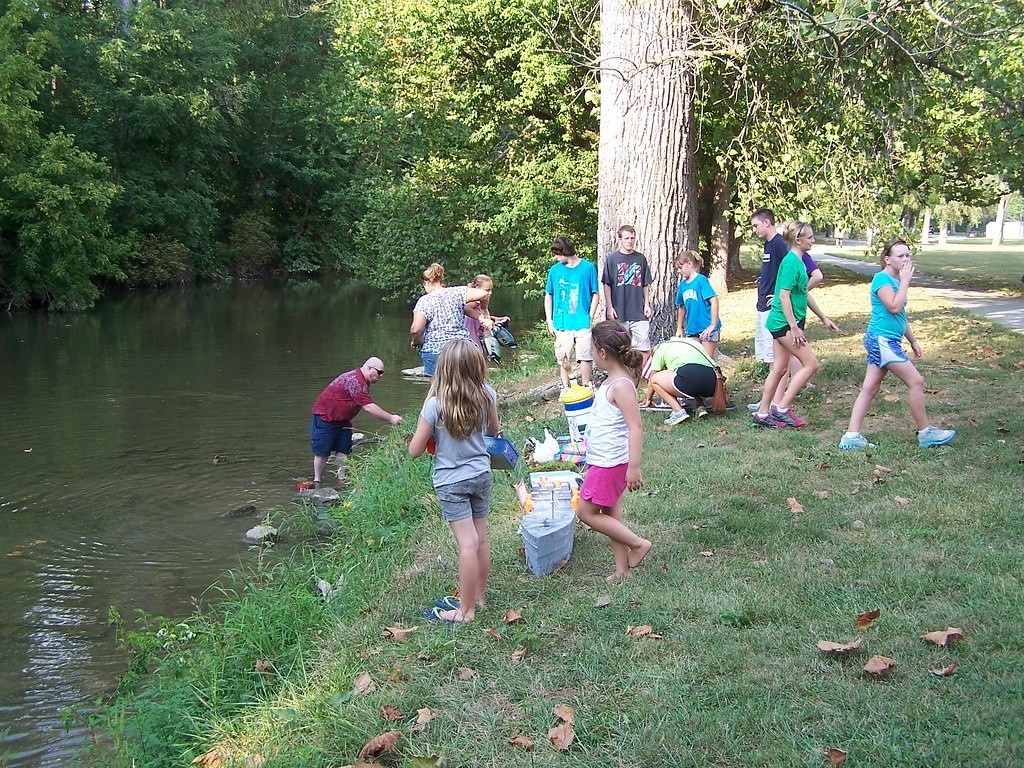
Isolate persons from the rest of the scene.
[410,263,492,390]
[408,339,499,625]
[788,250,825,397]
[460,274,511,361]
[753,220,842,430]
[837,238,958,450]
[748,207,790,415]
[543,236,601,403]
[639,336,719,426]
[673,251,723,362]
[311,355,405,489]
[556,289,571,314]
[577,318,654,585]
[601,225,654,378]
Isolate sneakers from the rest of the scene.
[916,425,956,448]
[747,401,762,411]
[751,412,786,428]
[769,406,805,427]
[694,406,708,418]
[558,383,571,402]
[584,380,598,398]
[664,409,690,426]
[839,433,877,450]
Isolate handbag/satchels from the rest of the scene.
[712,367,730,415]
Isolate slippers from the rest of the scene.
[422,607,454,623]
[434,595,461,611]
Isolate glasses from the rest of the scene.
[367,366,384,375]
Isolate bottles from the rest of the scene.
[561,372,596,445]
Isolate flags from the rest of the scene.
[641,333,663,379]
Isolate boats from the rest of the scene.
[520,470,577,575]
